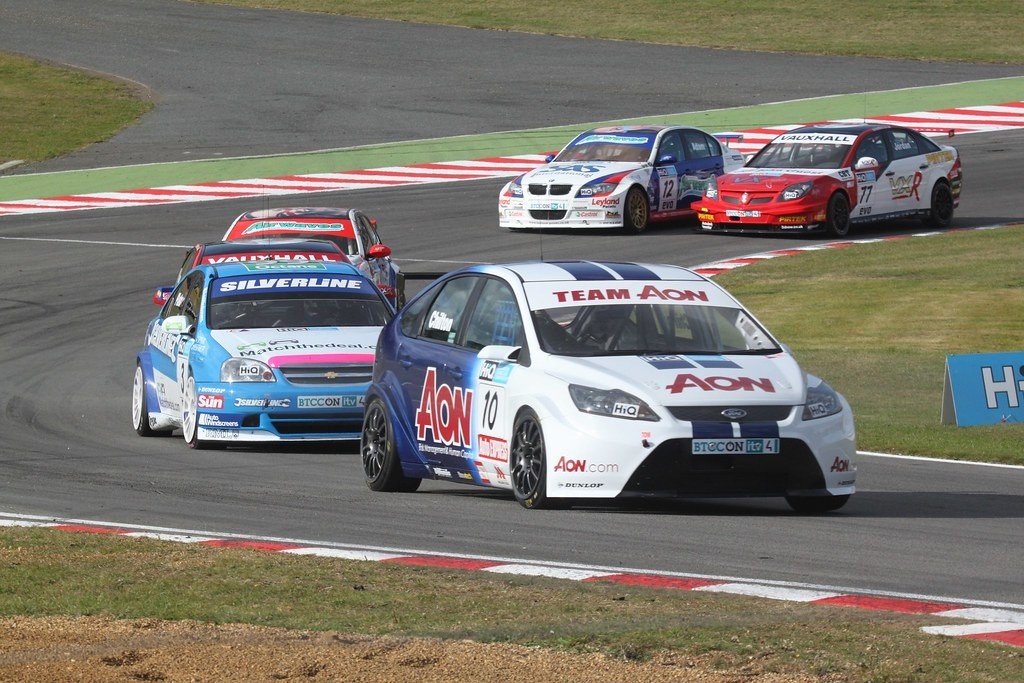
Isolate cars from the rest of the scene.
[691,121,962,239]
[498,125,747,233]
[153,237,397,306]
[222,207,398,312]
[360,261,858,511]
[132,259,399,450]
